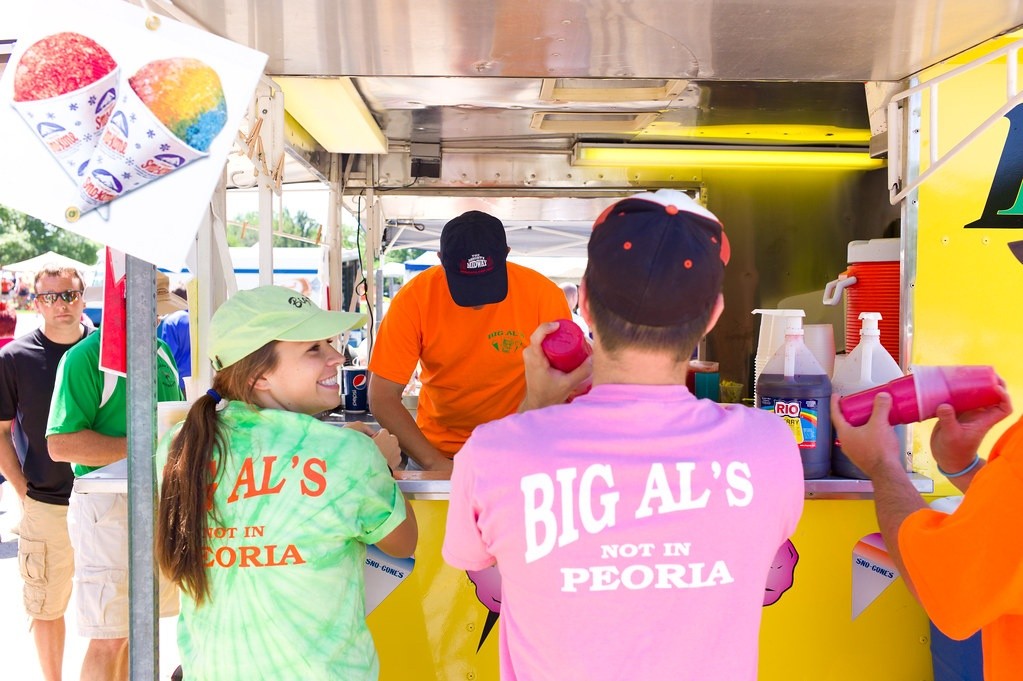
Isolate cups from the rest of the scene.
[542,320,592,373]
[910,363,998,423]
[686,360,745,403]
[752,308,836,408]
[342,366,369,412]
[839,366,952,428]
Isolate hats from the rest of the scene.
[587,189,730,331]
[440,210,508,307]
[155,272,188,317]
[206,286,367,371]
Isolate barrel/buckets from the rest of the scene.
[822,238,900,370]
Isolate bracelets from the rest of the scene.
[938,455,980,477]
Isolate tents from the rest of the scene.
[0,247,359,311]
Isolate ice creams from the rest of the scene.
[11,31,229,221]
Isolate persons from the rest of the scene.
[154,284,422,681]
[366,210,574,471]
[0,261,192,681]
[440,188,806,681]
[829,390,1023,681]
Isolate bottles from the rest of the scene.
[755,328,831,479]
[828,312,907,480]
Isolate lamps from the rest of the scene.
[272,77,388,154]
[571,142,887,171]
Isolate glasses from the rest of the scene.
[39,289,81,306]
